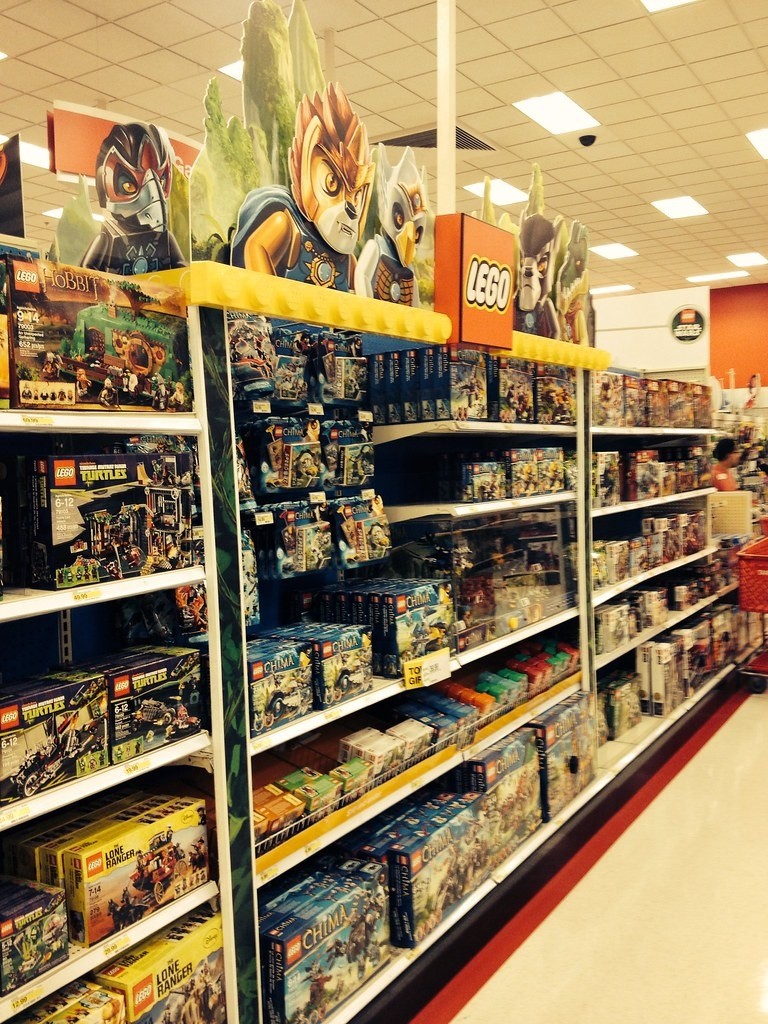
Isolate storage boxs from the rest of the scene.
[0,264,746,1024]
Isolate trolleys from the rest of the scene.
[752,501,768,536]
[737,536,768,692]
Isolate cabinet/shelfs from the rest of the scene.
[0,263,763,1024]
